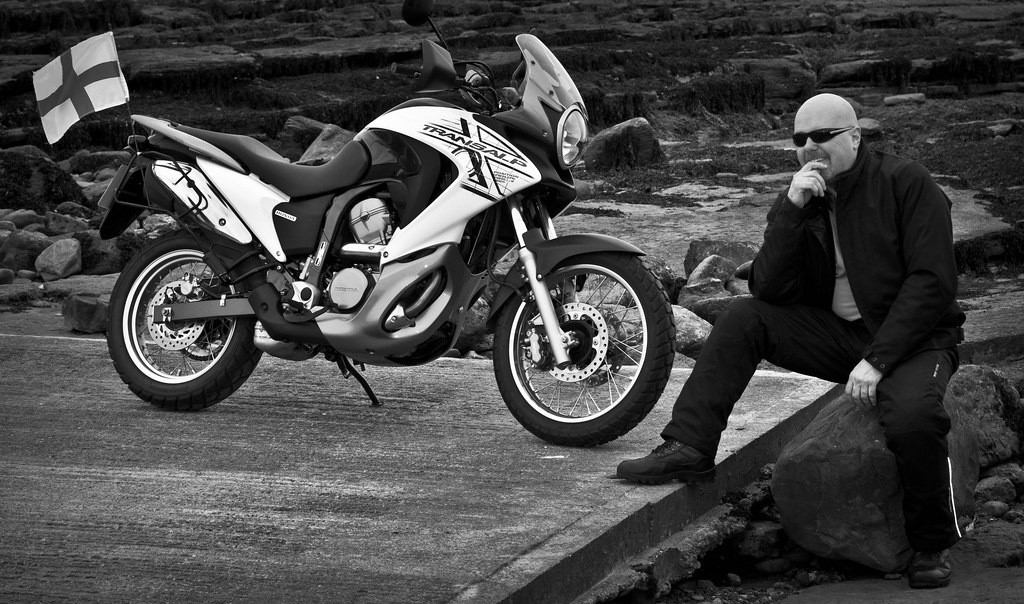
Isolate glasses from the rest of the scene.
[793,126,855,147]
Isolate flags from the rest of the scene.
[33,31,129,144]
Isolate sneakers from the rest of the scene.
[907,548,952,587]
[617,439,717,484]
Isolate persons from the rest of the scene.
[619,94,964,588]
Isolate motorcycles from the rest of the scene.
[92,0,678,451]
